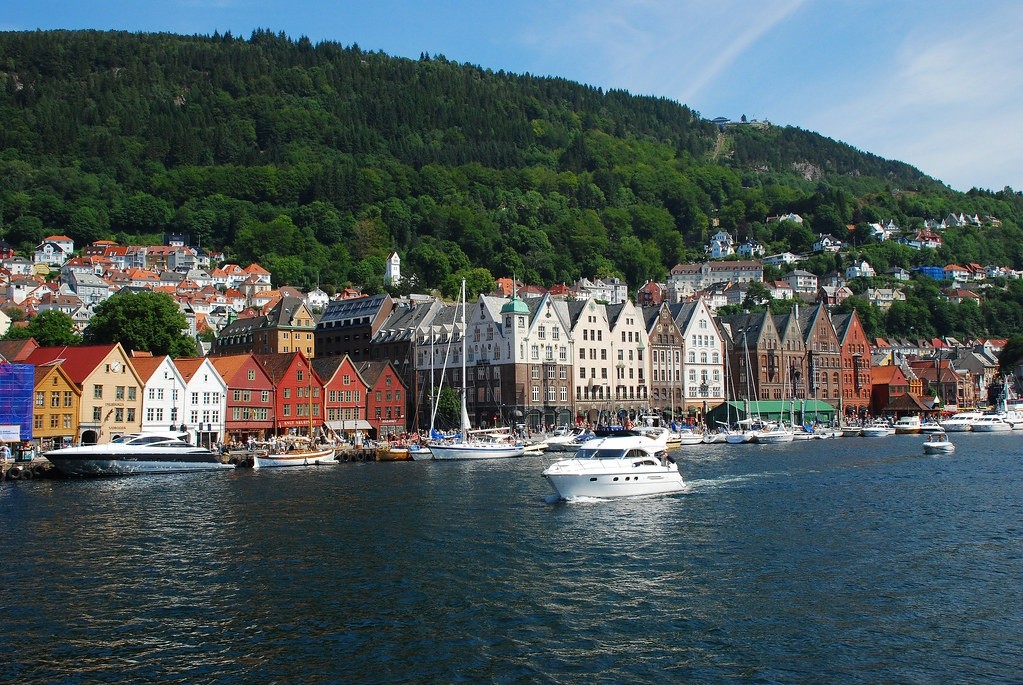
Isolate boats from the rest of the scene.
[893,414,945,434]
[862,420,895,436]
[816,423,844,439]
[540,406,685,500]
[41,431,235,477]
[922,432,955,455]
[664,420,727,447]
[841,422,862,436]
[940,408,1023,432]
[524,444,549,456]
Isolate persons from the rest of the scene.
[938,434,944,442]
[927,435,934,442]
[211,412,948,456]
[0,441,81,460]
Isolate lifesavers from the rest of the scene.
[21,469,33,480]
[230,458,240,466]
[365,454,372,462]
[348,454,357,462]
[357,453,364,461]
[33,466,46,479]
[240,460,248,468]
[341,453,349,462]
[7,468,21,480]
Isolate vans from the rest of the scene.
[554,426,568,436]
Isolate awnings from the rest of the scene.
[324,419,373,430]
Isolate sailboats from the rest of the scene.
[252,352,336,469]
[702,331,827,444]
[372,276,525,461]
